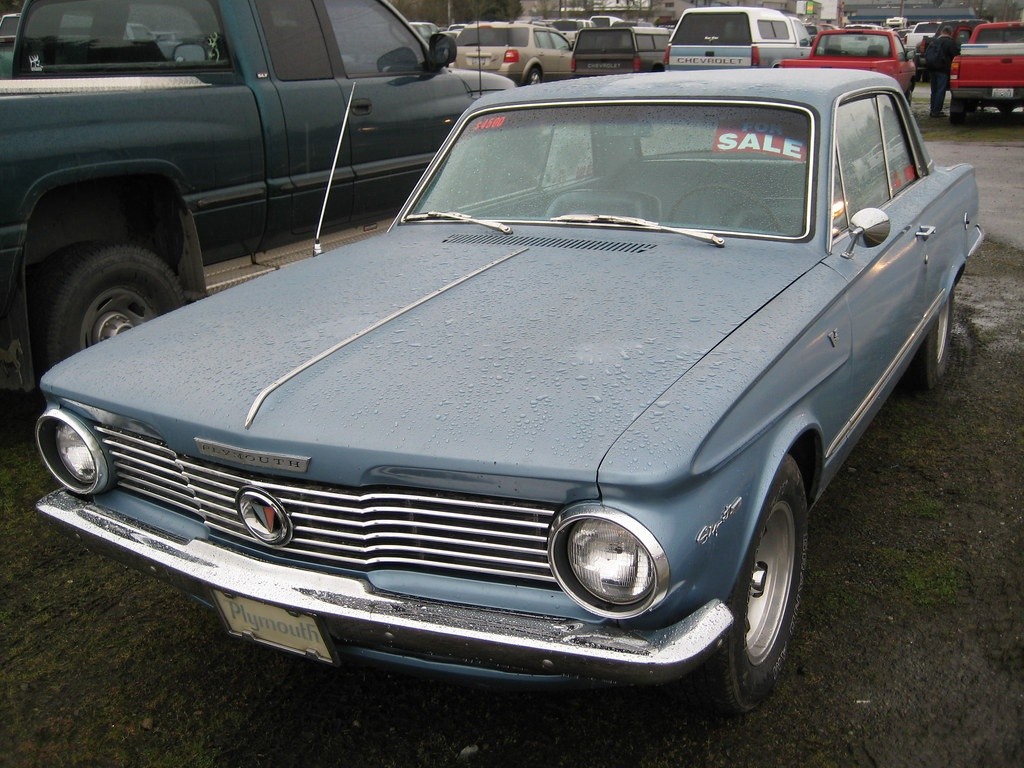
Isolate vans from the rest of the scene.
[664,7,814,72]
[570,28,675,79]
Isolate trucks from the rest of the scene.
[914,19,991,82]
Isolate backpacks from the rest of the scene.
[925,41,946,69]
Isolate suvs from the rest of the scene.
[448,20,573,87]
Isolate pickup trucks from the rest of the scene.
[867,134,905,171]
[946,22,1024,123]
[0,0,558,393]
[903,21,942,50]
[781,29,916,107]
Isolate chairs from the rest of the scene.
[866,44,885,57]
[824,43,842,54]
[548,188,663,224]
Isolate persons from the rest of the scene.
[925,25,961,118]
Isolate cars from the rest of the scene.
[0,13,185,59]
[886,16,916,42]
[589,16,622,28]
[820,24,837,29]
[840,24,889,57]
[806,24,823,45]
[408,21,468,39]
[550,20,594,45]
[34,69,984,714]
[612,20,653,28]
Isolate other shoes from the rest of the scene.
[930,111,947,118]
